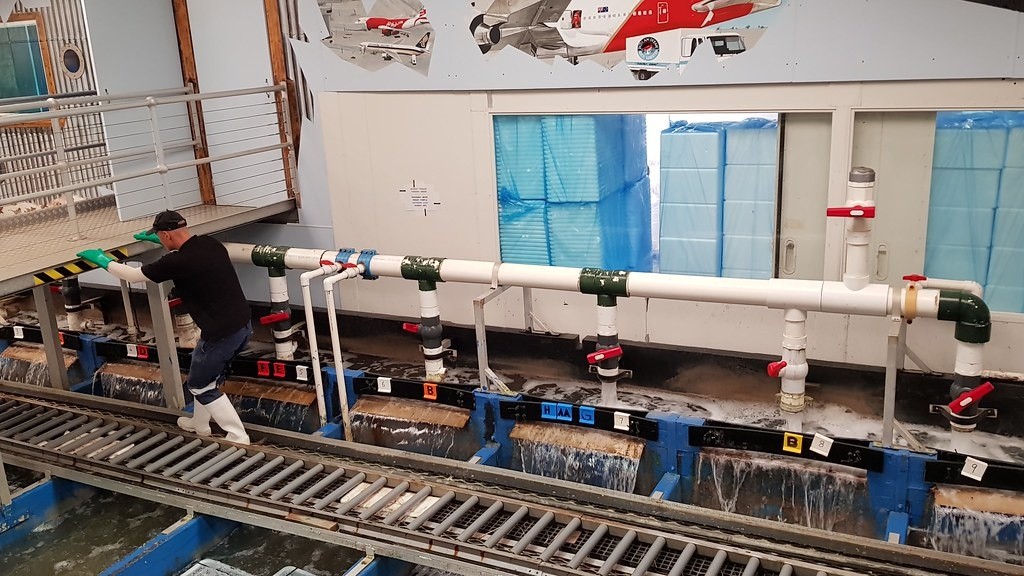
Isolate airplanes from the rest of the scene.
[357,32,432,65]
[345,8,428,38]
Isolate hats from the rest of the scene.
[146,210,187,238]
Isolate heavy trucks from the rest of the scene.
[626,27,747,80]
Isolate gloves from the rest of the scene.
[76,248,115,271]
[134,230,160,244]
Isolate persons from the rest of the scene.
[76,209,251,445]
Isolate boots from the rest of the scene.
[202,392,250,445]
[177,396,211,436]
